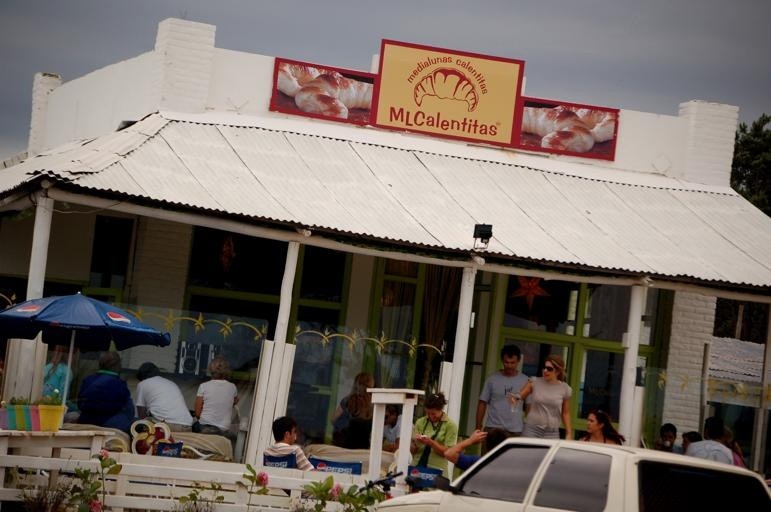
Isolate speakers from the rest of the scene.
[178,340,201,375]
[206,344,226,377]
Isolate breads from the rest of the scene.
[553,105,615,143]
[277,62,328,97]
[520,106,594,153]
[294,71,374,119]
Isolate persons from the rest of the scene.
[43,340,74,407]
[653,422,684,455]
[193,354,241,456]
[410,392,459,490]
[679,429,705,453]
[75,350,136,432]
[331,372,376,450]
[262,415,316,474]
[685,415,735,466]
[135,360,194,433]
[383,405,418,454]
[719,424,749,467]
[509,353,574,442]
[444,426,490,471]
[474,342,530,458]
[578,407,626,446]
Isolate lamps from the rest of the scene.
[635,362,669,392]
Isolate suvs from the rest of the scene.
[367,436,771,511]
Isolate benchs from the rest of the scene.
[305,441,396,474]
[64,420,134,453]
[160,432,232,461]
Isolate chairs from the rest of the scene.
[406,461,443,488]
[154,441,184,459]
[262,450,298,471]
[308,456,367,474]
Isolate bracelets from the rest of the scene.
[53,360,58,367]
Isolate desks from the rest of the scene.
[0,425,117,495]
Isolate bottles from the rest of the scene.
[510,390,523,413]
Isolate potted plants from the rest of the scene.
[0,387,72,437]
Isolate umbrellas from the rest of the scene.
[1,290,173,431]
